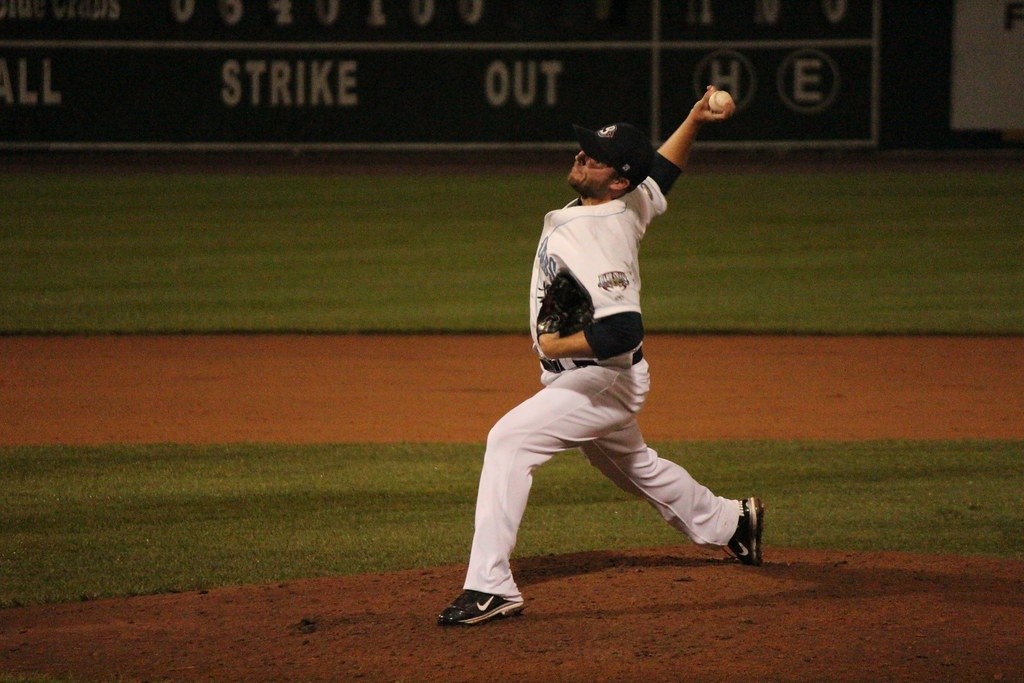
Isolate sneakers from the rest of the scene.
[727,495,763,568]
[436,590,523,624]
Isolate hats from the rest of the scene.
[572,123,655,185]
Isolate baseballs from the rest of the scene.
[709,91,735,114]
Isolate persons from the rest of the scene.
[442,86,764,623]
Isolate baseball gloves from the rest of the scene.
[531,270,593,356]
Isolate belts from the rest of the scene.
[537,347,644,374]
